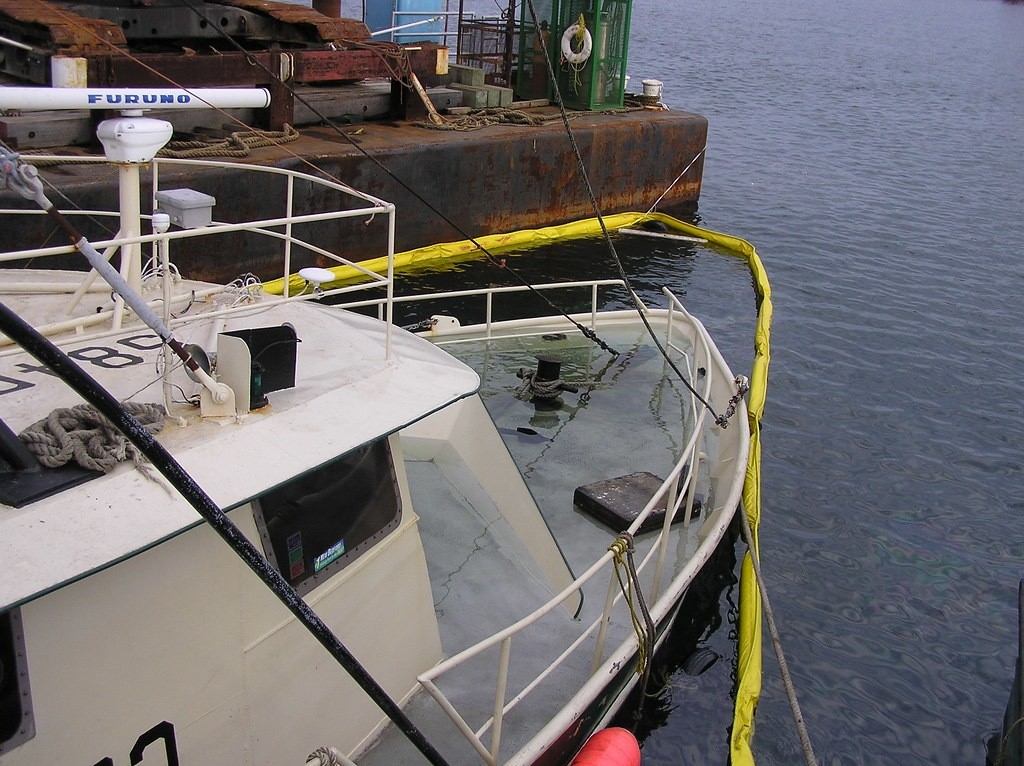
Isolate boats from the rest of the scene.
[1,88,753,766]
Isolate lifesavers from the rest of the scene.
[561,25,592,62]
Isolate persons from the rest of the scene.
[533,20,550,91]
